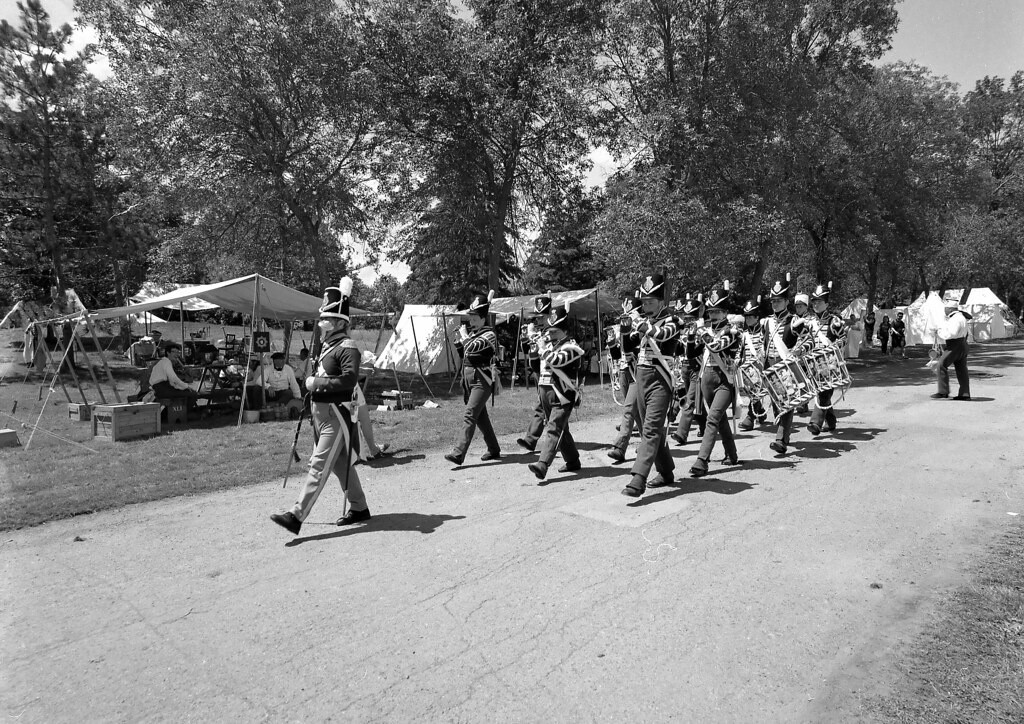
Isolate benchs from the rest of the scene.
[127,395,187,425]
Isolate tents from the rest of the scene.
[841,288,1014,344]
[0,274,396,448]
[376,286,620,390]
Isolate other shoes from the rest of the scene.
[931,393,947,399]
[954,394,970,400]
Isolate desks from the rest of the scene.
[184,365,238,420]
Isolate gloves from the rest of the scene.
[537,339,546,350]
[528,323,535,335]
[620,313,632,327]
[459,324,468,336]
[305,376,316,391]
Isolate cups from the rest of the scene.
[218,356,224,364]
[205,353,210,360]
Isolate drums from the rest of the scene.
[760,355,818,414]
[738,354,775,404]
[799,343,851,394]
[607,353,620,375]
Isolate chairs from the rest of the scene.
[218,334,235,356]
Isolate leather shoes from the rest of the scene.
[337,508,371,526]
[690,458,709,475]
[647,472,674,488]
[270,512,301,535]
[608,405,836,466]
[445,439,581,479]
[622,474,647,496]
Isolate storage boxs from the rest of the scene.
[382,392,412,411]
[91,402,161,443]
[68,401,96,421]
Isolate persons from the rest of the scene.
[889,312,908,358]
[930,302,971,400]
[152,330,162,346]
[607,273,846,499]
[270,277,371,535]
[149,344,201,420]
[231,353,264,399]
[251,352,302,417]
[877,316,892,355]
[845,314,863,358]
[518,290,585,480]
[296,349,312,385]
[444,289,501,464]
[863,311,876,345]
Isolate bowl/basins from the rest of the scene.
[243,411,260,423]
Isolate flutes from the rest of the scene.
[455,326,475,331]
[689,318,717,325]
[620,311,653,319]
[527,339,557,345]
[522,324,543,329]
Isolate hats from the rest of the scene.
[545,296,571,331]
[623,275,830,317]
[467,289,496,315]
[320,276,353,323]
[945,301,958,308]
[531,290,552,317]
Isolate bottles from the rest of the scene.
[260,404,280,420]
